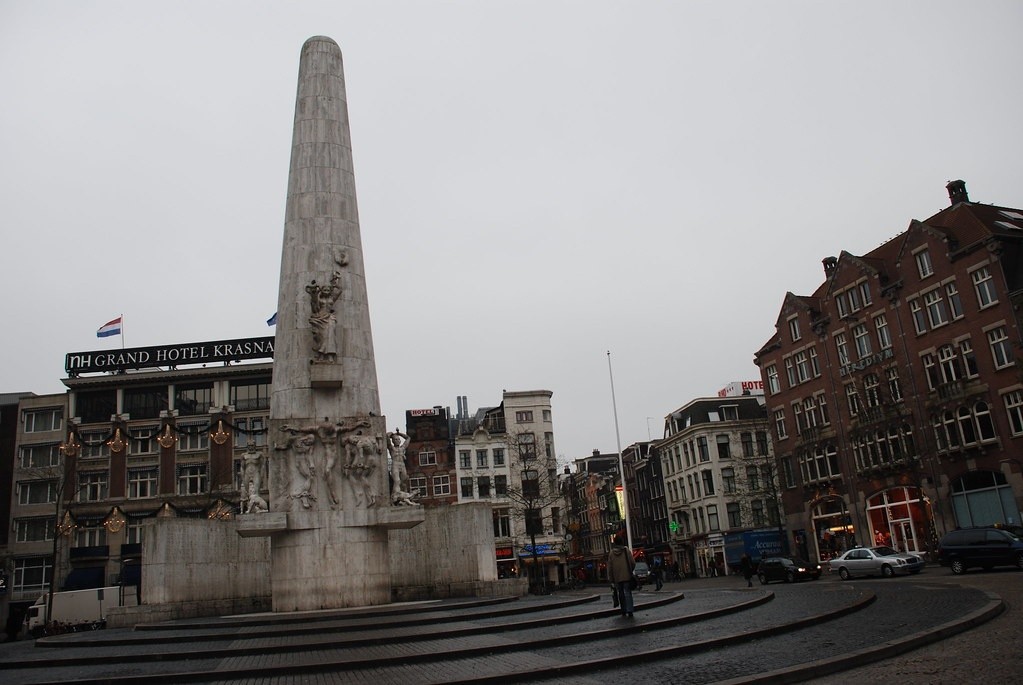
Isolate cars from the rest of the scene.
[827,546,927,581]
[757,555,822,583]
[634,563,653,585]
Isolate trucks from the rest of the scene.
[25,586,120,632]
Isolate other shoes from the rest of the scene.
[628,613,633,617]
[622,611,626,616]
[748,582,752,587]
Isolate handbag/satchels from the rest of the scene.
[612,586,619,608]
[630,573,638,591]
[749,563,753,570]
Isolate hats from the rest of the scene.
[613,536,623,545]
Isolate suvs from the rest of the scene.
[936,527,1023,575]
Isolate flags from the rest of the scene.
[97,317,121,338]
[266,312,277,327]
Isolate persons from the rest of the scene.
[820,527,854,550]
[708,557,718,578]
[740,554,753,587]
[875,527,891,546]
[607,535,636,617]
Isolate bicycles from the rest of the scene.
[32,618,107,638]
